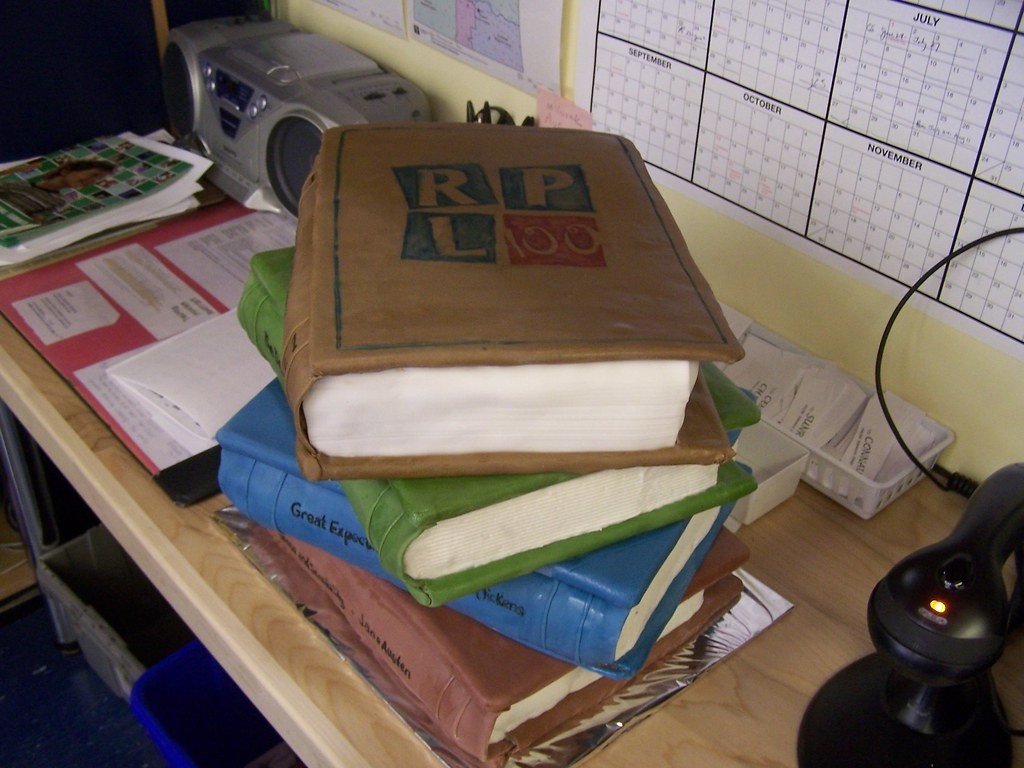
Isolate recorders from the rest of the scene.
[163,11,432,223]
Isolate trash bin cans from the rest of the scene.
[36,523,198,703]
[129,639,306,768]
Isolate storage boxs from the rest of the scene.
[34,519,300,768]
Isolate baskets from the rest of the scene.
[708,321,954,518]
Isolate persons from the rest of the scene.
[0,160,115,218]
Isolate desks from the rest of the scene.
[1,146,1024,768]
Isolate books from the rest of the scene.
[269,525,752,762]
[283,123,746,481]
[217,379,760,677]
[237,247,760,607]
